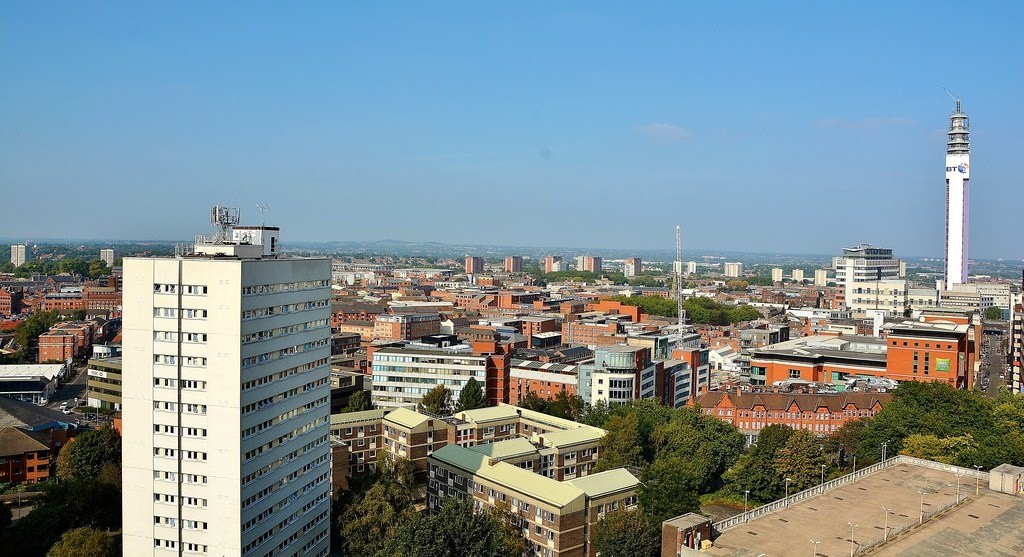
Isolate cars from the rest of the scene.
[60,402,101,421]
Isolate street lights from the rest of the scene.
[954,473,963,504]
[847,522,859,557]
[786,478,791,507]
[974,464,983,496]
[882,445,887,470]
[745,491,750,523]
[917,490,928,524]
[810,539,820,557]
[822,465,826,486]
[882,506,892,542]
